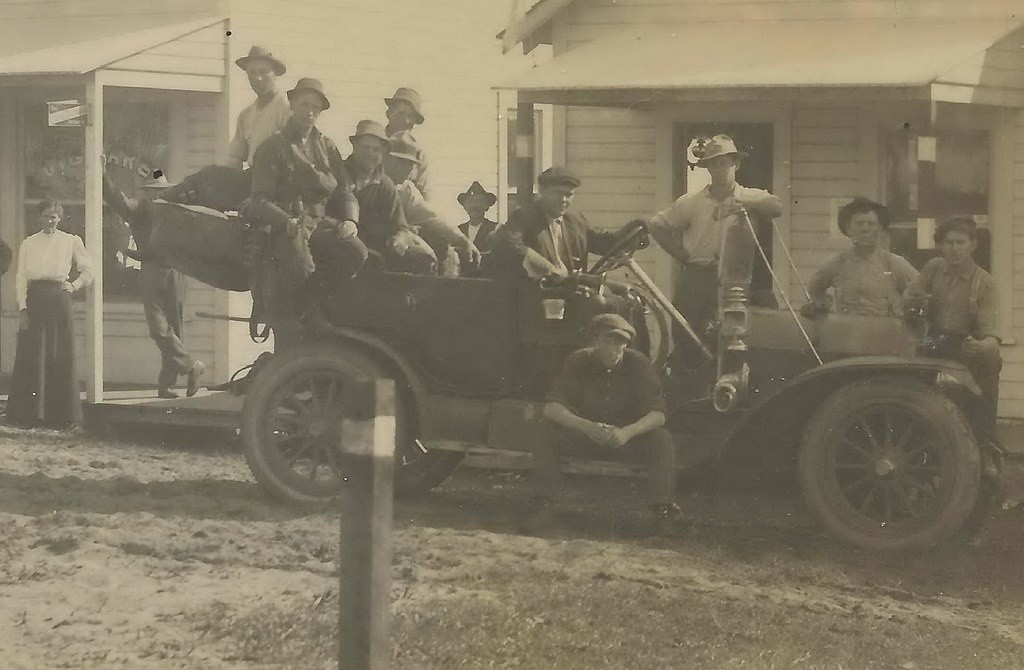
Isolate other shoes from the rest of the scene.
[187,361,204,397]
[983,435,1009,456]
[657,504,675,535]
[66,421,81,434]
[156,384,178,399]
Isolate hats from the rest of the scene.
[384,87,425,123]
[838,196,893,237]
[348,119,393,155]
[286,79,329,110]
[386,138,421,165]
[695,134,748,168]
[458,182,497,207]
[539,167,580,189]
[587,313,638,343]
[234,45,286,76]
[136,170,178,189]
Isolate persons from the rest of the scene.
[494,167,649,288]
[102,153,206,398]
[646,134,783,368]
[530,313,683,532]
[124,46,293,270]
[902,218,1004,478]
[457,180,503,251]
[5,199,95,432]
[247,77,482,337]
[801,195,920,318]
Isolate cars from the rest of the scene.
[199,222,985,562]
